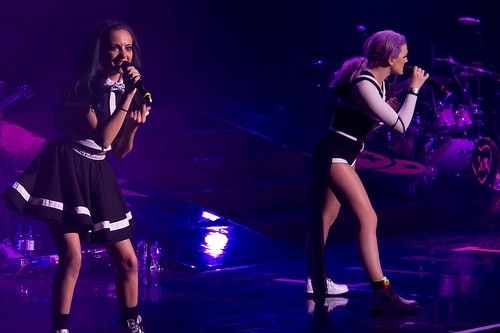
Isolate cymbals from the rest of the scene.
[435,55,460,65]
[463,62,493,74]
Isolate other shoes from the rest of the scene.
[121,315,146,333]
[369,276,419,313]
[306,277,350,296]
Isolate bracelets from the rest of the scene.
[409,87,419,94]
[118,107,127,113]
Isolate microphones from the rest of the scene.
[405,65,445,91]
[120,61,152,103]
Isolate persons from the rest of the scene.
[305,30,429,312]
[4,20,151,333]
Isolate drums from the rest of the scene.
[433,140,477,173]
[438,106,474,132]
